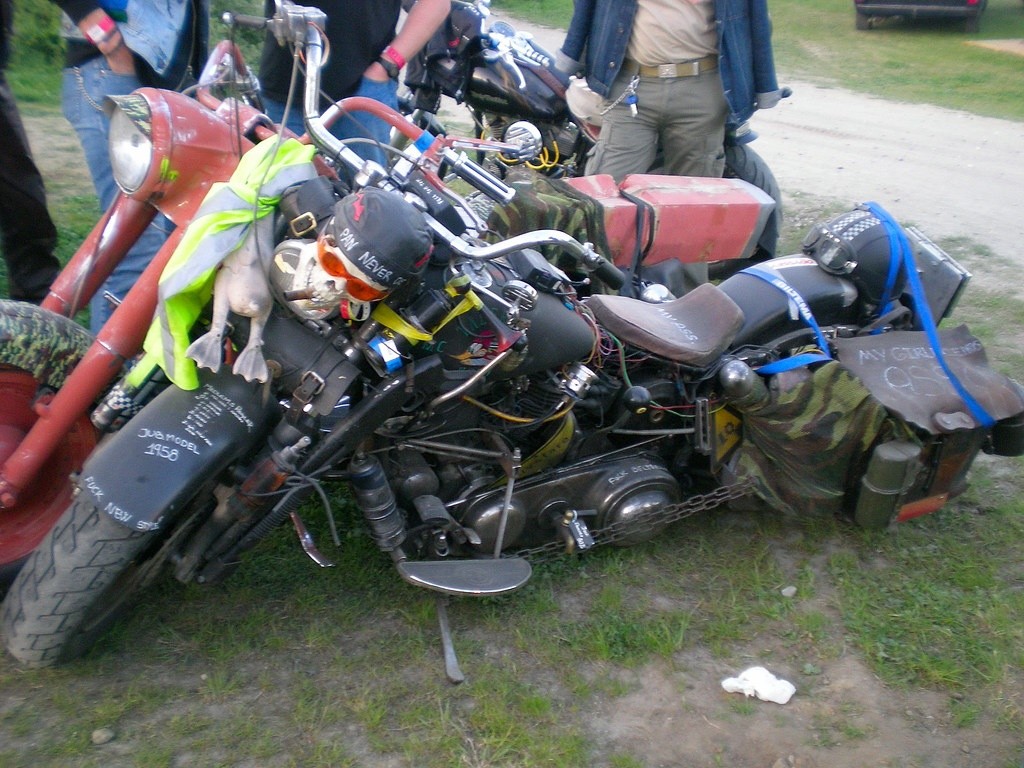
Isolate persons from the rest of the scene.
[49,0,210,374]
[555,0,784,297]
[0,0,64,305]
[258,1,453,186]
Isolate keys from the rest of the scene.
[627,94,638,117]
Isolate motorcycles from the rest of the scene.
[0,0,972,672]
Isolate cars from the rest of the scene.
[852,0,989,32]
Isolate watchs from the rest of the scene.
[374,56,400,78]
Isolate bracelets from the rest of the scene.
[381,44,407,71]
[84,14,116,50]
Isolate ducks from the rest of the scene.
[185,208,273,383]
